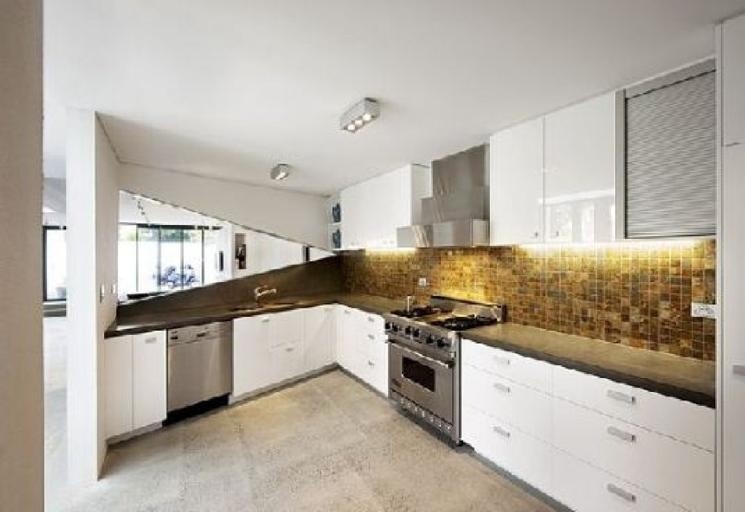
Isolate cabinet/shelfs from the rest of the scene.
[488,91,614,246]
[229,303,338,404]
[337,305,357,378]
[105,330,167,445]
[550,363,715,512]
[460,339,549,503]
[340,165,431,250]
[357,310,388,398]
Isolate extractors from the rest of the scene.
[394,145,490,252]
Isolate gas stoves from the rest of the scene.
[383,303,498,352]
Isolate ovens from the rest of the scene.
[385,332,460,446]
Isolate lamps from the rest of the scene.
[339,98,378,134]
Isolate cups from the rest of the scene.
[404,295,414,312]
[414,285,430,308]
